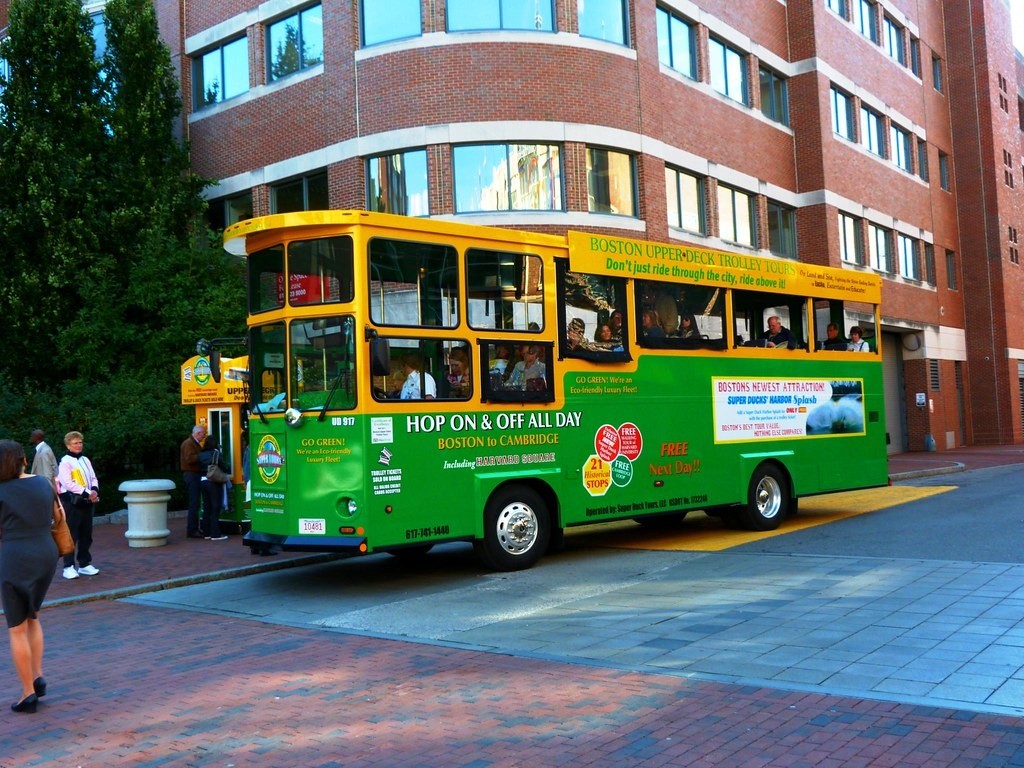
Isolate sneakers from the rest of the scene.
[63,565,80,580]
[78,564,100,576]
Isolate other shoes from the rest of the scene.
[187,528,204,539]
[204,533,229,540]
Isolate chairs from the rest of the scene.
[701,334,823,350]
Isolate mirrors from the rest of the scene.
[901,332,921,351]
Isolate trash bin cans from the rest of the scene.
[927,438,936,452]
[117,478,177,547]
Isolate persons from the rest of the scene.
[529,322,539,331]
[757,316,797,350]
[27,430,59,479]
[48,432,99,580]
[639,309,703,339]
[180,425,207,538]
[197,435,230,540]
[447,347,470,399]
[242,443,251,504]
[567,310,623,352]
[491,342,547,392]
[1,440,66,712]
[846,326,869,352]
[399,352,436,399]
[819,323,847,350]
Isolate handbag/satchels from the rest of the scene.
[206,449,227,483]
[46,477,76,556]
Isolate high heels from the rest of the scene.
[33,677,46,698]
[12,690,38,713]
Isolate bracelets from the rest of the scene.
[852,347,854,351]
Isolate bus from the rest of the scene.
[222,210,892,568]
